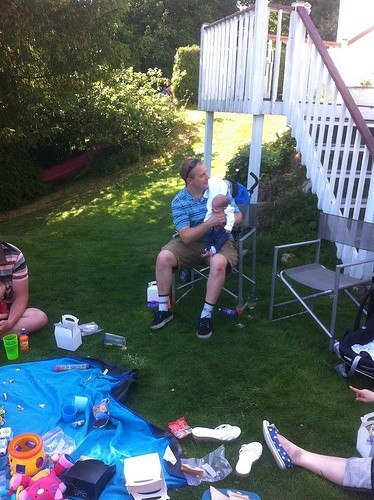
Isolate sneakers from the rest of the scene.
[197,312,213,338]
[150,311,173,330]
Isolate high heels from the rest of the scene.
[236,442,263,478]
[192,424,241,443]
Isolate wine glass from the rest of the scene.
[235,299,246,328]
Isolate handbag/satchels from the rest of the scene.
[53,314,82,351]
[357,412,374,458]
[64,459,116,500]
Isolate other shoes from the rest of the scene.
[263,420,294,470]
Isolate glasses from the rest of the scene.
[185,158,201,179]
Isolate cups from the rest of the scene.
[2,333,19,361]
[102,332,127,348]
[72,395,88,412]
[62,404,78,423]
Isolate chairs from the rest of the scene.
[171,202,259,328]
[270,210,374,353]
[64,457,117,500]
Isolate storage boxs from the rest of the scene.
[146,280,160,304]
[123,452,170,500]
[54,314,83,352]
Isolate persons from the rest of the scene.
[0,283,9,320]
[201,194,235,257]
[0,241,48,342]
[263,386,374,492]
[150,158,243,338]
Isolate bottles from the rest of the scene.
[331,339,342,359]
[54,363,90,371]
[19,328,29,352]
[218,307,237,316]
[8,433,46,478]
